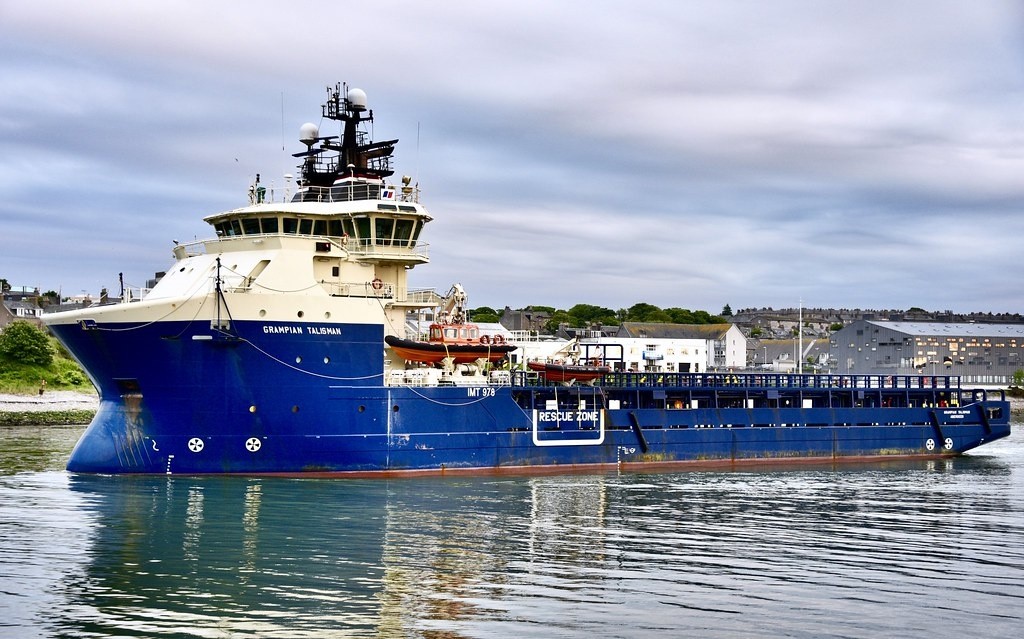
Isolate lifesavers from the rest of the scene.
[342,233,348,245]
[923,377,928,385]
[372,277,383,289]
[480,334,490,343]
[494,334,503,344]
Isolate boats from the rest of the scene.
[527,360,613,383]
[28,78,1015,482]
[384,311,519,367]
